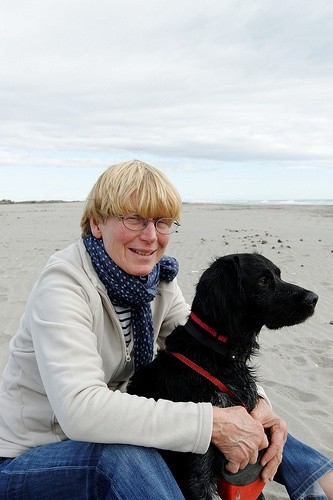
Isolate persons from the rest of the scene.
[0,159,333,500]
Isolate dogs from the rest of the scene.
[114,255,320,500]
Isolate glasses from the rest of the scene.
[112,213,182,235]
